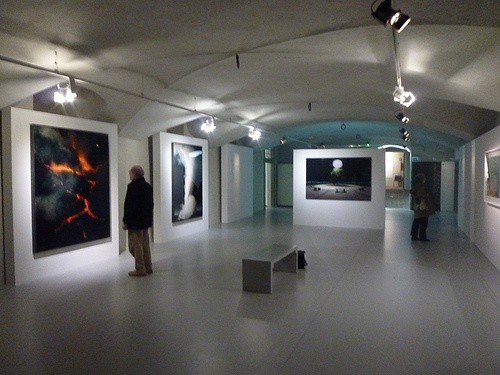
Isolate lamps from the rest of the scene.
[393,87,416,141]
[370,0,412,33]
[279,137,287,145]
[58,76,78,103]
[205,117,215,130]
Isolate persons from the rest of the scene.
[123,166,154,275]
[411,173,434,241]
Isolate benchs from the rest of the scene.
[241,243,298,294]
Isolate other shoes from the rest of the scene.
[146,270,152,273]
[411,237,419,241]
[420,237,430,242]
[128,271,146,276]
